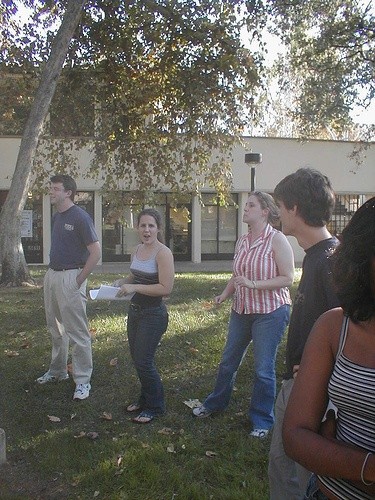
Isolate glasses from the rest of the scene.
[48,187,70,192]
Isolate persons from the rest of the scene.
[34,173,102,400]
[265,166,341,500]
[192,191,296,438]
[282,195,375,500]
[111,208,174,425]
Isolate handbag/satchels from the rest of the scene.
[268,378,319,500]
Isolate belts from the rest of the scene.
[129,303,161,311]
[49,267,84,271]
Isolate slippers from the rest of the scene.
[132,411,157,423]
[127,403,144,412]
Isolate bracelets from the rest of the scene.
[253,281,256,288]
[361,452,374,486]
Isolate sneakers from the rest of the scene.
[193,404,215,418]
[249,429,270,438]
[72,384,91,401]
[35,370,70,385]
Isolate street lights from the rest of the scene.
[244,153,262,192]
